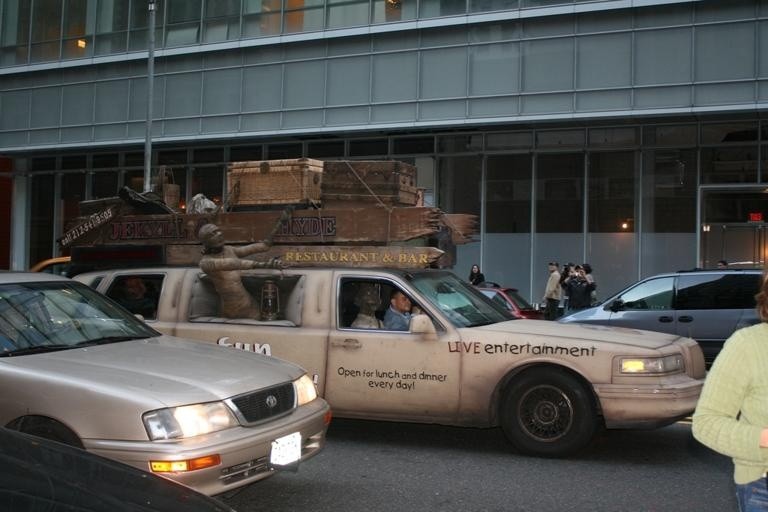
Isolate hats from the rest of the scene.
[549,261,559,266]
[563,262,576,267]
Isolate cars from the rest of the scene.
[1,268,332,498]
[68,267,710,459]
[470,280,546,320]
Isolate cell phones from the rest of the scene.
[575,272,579,276]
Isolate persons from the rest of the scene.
[350,284,384,329]
[383,289,421,330]
[199,223,279,320]
[0,297,76,352]
[690,271,768,511]
[717,260,728,269]
[118,275,156,318]
[469,265,485,285]
[542,261,598,321]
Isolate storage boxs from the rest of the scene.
[224,155,420,211]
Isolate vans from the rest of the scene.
[553,269,764,371]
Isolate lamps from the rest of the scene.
[261,278,282,321]
